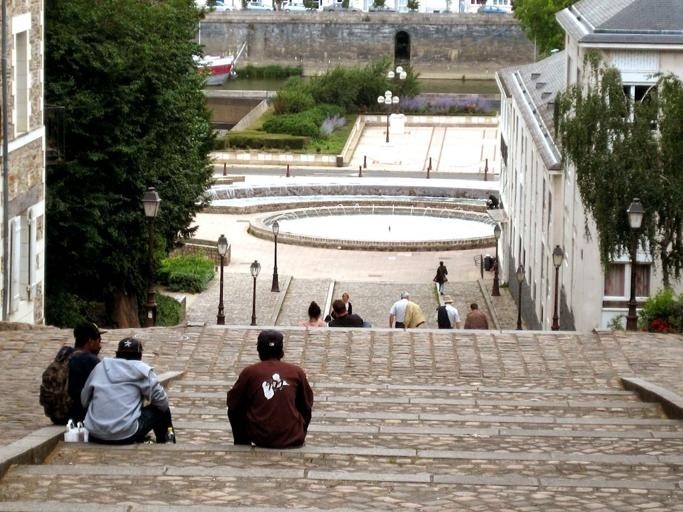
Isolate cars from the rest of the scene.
[245,2,273,14]
[323,3,361,14]
[477,6,506,14]
[369,4,396,13]
[202,2,233,12]
[282,2,310,13]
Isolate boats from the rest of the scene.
[191,41,247,87]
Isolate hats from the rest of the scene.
[75,322,108,339]
[256,330,283,346]
[117,338,143,353]
[444,296,454,303]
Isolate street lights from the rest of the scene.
[490,223,502,296]
[248,259,260,325]
[550,245,564,331]
[141,186,161,326]
[625,197,646,331]
[216,233,229,325]
[386,66,409,113]
[515,263,524,330]
[270,220,281,291]
[376,90,399,142]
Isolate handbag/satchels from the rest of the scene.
[63,420,89,443]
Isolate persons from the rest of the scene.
[298,290,493,329]
[36,322,107,428]
[225,329,316,449]
[76,339,177,446]
[485,190,500,209]
[433,260,450,295]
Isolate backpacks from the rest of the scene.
[40,346,82,420]
